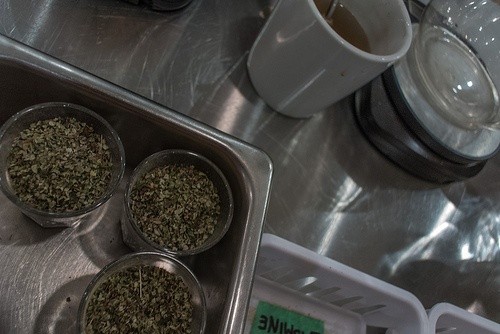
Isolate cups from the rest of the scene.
[247,0,413,121]
[410,0,500,138]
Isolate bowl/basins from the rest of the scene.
[0,102,127,226]
[120,149,233,257]
[77,251,207,334]
